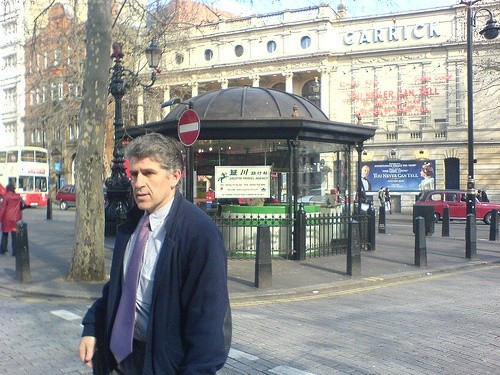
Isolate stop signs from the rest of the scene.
[177,108,200,147]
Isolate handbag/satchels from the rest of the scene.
[20,198,26,210]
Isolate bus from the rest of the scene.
[0,145,49,209]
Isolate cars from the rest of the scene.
[55,184,77,210]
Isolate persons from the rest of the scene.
[482,191,490,202]
[477,190,482,202]
[419,164,434,190]
[79,132,233,375]
[0,183,26,256]
[378,186,385,206]
[385,188,391,213]
[460,194,466,201]
[360,165,372,191]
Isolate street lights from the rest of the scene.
[459,1,500,216]
[104,35,162,240]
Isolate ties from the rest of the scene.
[109,214,151,363]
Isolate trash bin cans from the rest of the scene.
[413,205,435,236]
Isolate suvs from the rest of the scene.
[415,188,500,225]
